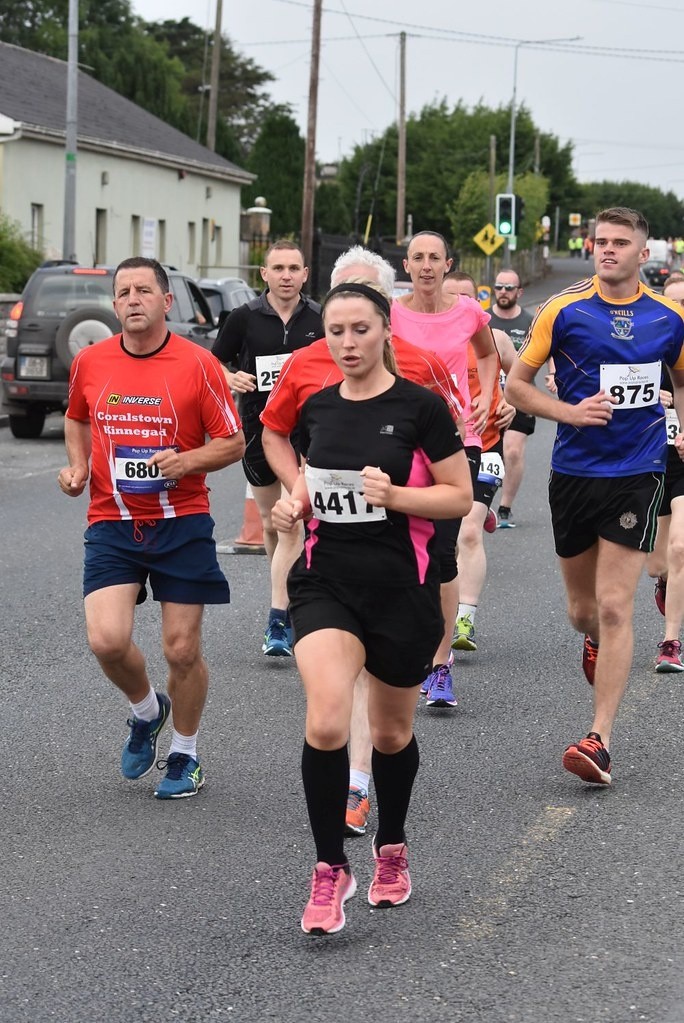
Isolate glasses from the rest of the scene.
[494,283,515,291]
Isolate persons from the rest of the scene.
[58,255,246,800]
[647,277,684,671]
[568,233,684,272]
[272,275,475,934]
[212,231,557,838]
[503,207,684,787]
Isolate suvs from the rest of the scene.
[196,276,262,324]
[0,259,232,439]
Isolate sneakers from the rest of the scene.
[582,634,599,685]
[302,861,358,936]
[483,508,498,533]
[262,618,294,656]
[368,835,412,907]
[120,692,172,779]
[562,732,612,784]
[654,576,667,616]
[153,752,204,800]
[345,786,369,837]
[426,663,458,707]
[655,639,684,673]
[420,649,454,693]
[497,512,516,528]
[452,613,478,651]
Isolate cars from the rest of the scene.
[643,260,670,287]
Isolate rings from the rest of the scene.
[484,421,486,424]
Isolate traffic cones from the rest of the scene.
[215,481,267,556]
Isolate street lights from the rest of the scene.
[507,35,582,194]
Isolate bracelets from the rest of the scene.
[549,373,555,375]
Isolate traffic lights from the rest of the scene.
[515,195,525,235]
[496,193,515,237]
